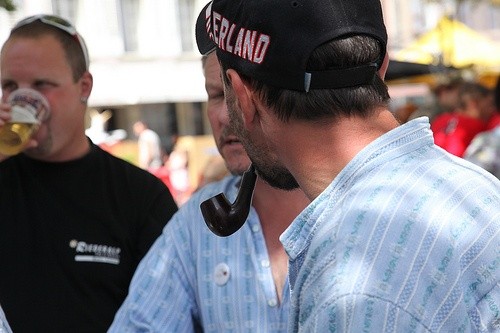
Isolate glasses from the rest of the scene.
[10,13,91,73]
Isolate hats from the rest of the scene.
[212,0,388,93]
[195,0,219,57]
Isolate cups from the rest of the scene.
[0,87,51,155]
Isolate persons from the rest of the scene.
[106,48,312,333]
[408,72,500,177]
[132,120,190,203]
[195,0,500,333]
[0,14,178,333]
